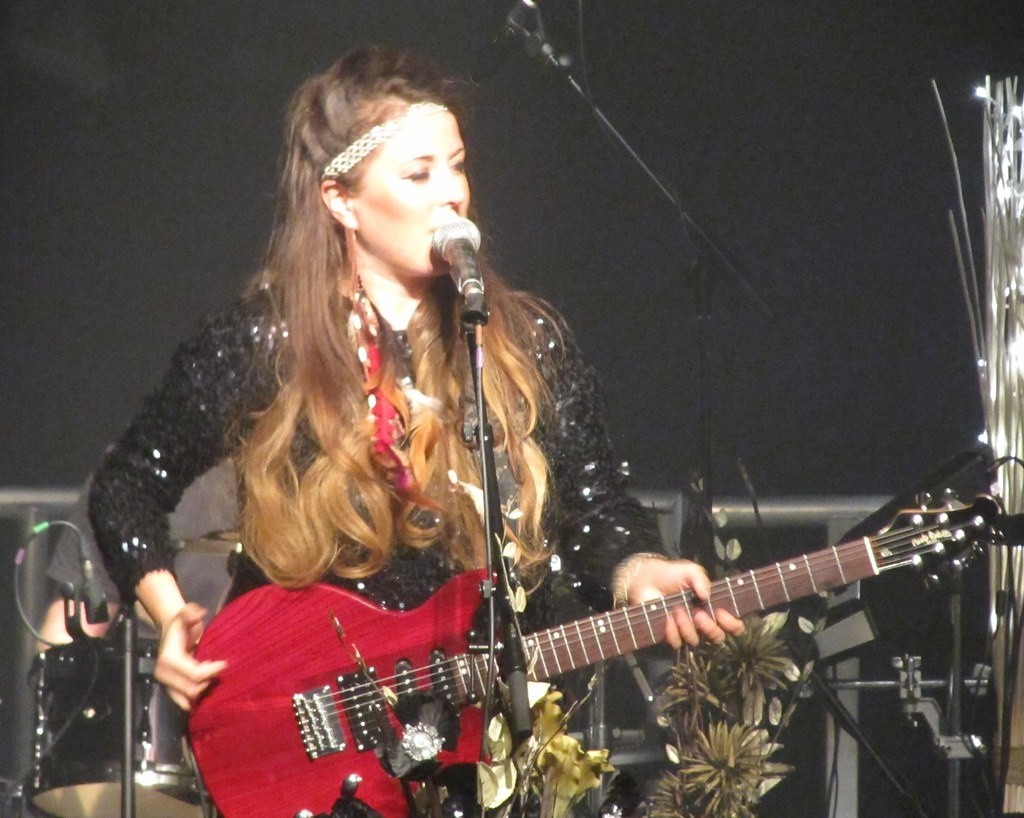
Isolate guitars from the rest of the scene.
[188,493,1002,818]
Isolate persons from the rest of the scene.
[38,438,240,817]
[88,45,744,817]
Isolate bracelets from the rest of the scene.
[614,554,667,612]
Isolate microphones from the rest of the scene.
[469,0,539,86]
[76,534,111,625]
[432,216,485,311]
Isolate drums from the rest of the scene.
[30,638,209,818]
